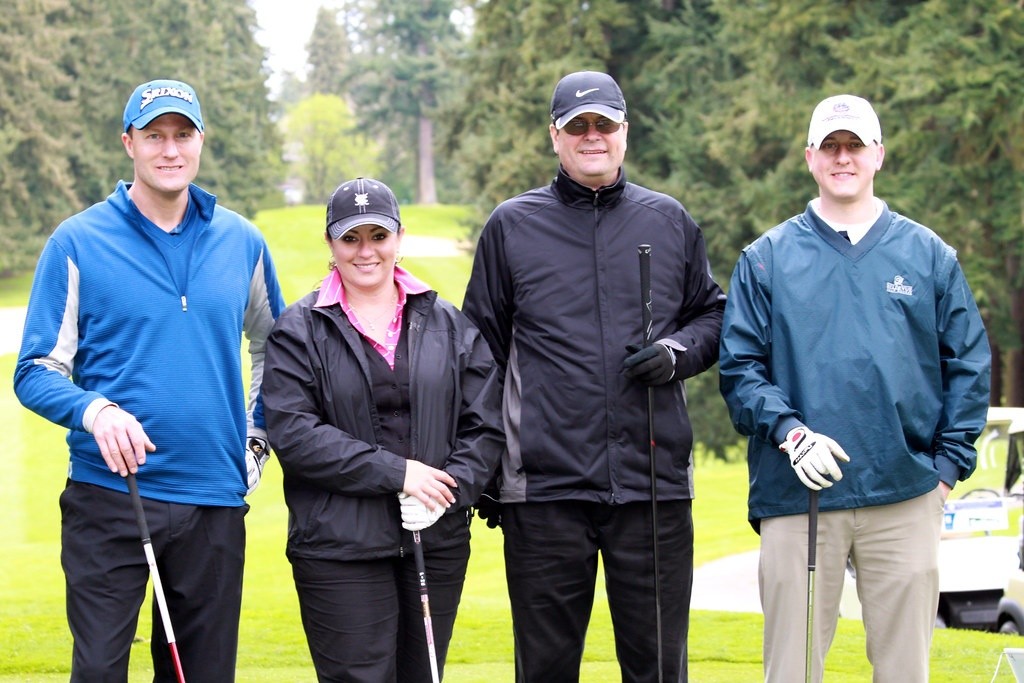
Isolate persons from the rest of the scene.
[12,78,288,682]
[264,176,503,683]
[458,71,727,683]
[720,94,992,683]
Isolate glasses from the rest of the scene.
[561,116,619,136]
[821,140,864,154]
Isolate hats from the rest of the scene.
[326,175,400,242]
[807,94,882,148]
[123,79,204,136]
[550,71,627,130]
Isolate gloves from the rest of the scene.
[244,437,270,499]
[396,487,447,531]
[473,459,503,529]
[623,341,673,387]
[778,427,851,490]
